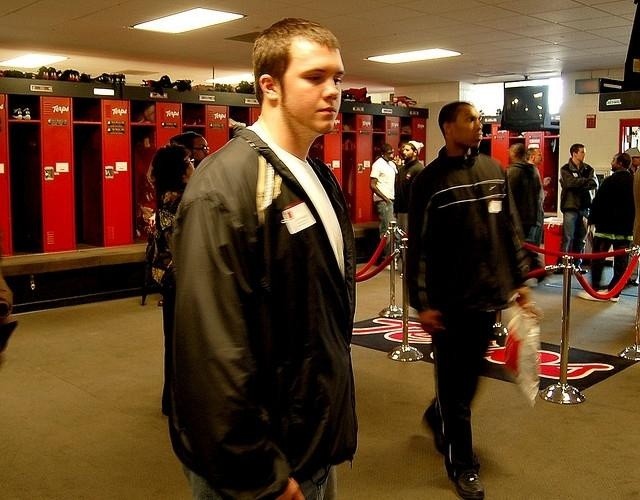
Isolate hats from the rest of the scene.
[626,148,640,157]
[408,140,424,153]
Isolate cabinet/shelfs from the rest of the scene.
[0,78,429,263]
[472,114,561,218]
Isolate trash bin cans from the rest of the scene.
[543,216,563,267]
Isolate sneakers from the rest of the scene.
[576,288,618,301]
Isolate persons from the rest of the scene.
[526,147,552,187]
[395,140,425,279]
[406,103,533,499]
[576,152,634,301]
[170,131,211,168]
[161,17,358,500]
[505,143,551,283]
[369,143,401,271]
[137,144,196,416]
[625,147,640,287]
[554,143,600,275]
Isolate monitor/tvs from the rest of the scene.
[504,85,551,131]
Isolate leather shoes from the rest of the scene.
[457,473,484,499]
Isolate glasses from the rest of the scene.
[191,146,211,154]
